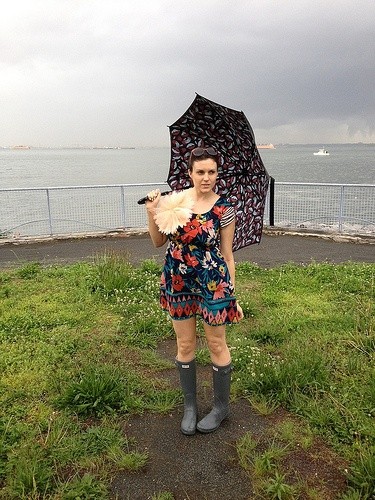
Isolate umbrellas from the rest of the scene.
[165,92,271,254]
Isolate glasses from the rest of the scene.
[189,147,217,166]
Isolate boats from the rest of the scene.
[257,144,275,149]
[313,149,330,156]
[14,145,30,150]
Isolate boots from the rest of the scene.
[197,363,232,433]
[176,355,199,434]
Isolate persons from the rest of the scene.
[142,146,245,437]
